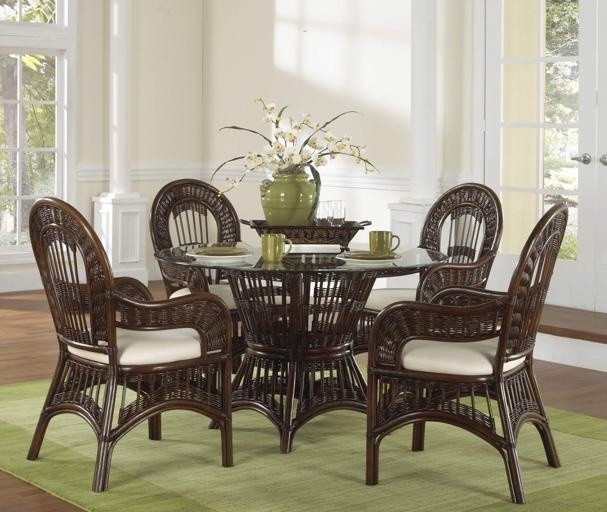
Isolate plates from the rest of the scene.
[336,251,402,264]
[185,247,253,262]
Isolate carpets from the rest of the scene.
[0,366,607,512]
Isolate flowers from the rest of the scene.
[211,98,379,206]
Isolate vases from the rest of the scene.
[260,174,318,224]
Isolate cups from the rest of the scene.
[369,230,400,257]
[261,233,292,263]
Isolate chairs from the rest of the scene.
[366,203,570,503]
[29,198,234,494]
[353,183,503,354]
[150,180,291,374]
